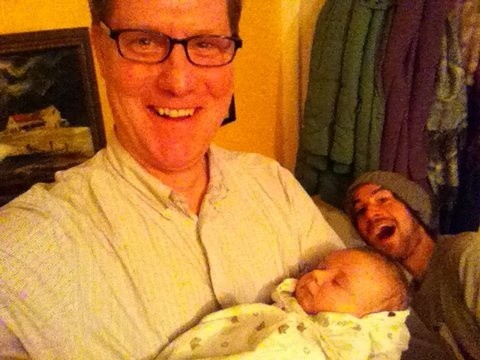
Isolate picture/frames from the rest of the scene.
[0,27,107,207]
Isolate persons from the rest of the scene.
[344,169,480,359]
[0,0,416,360]
[152,246,413,360]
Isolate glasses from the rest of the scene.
[97,18,244,70]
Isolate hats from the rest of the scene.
[344,171,432,226]
